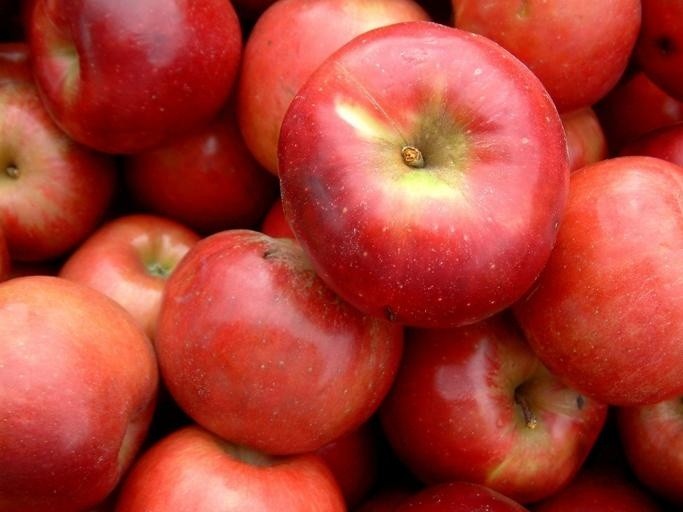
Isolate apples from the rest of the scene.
[1,0,683,512]
